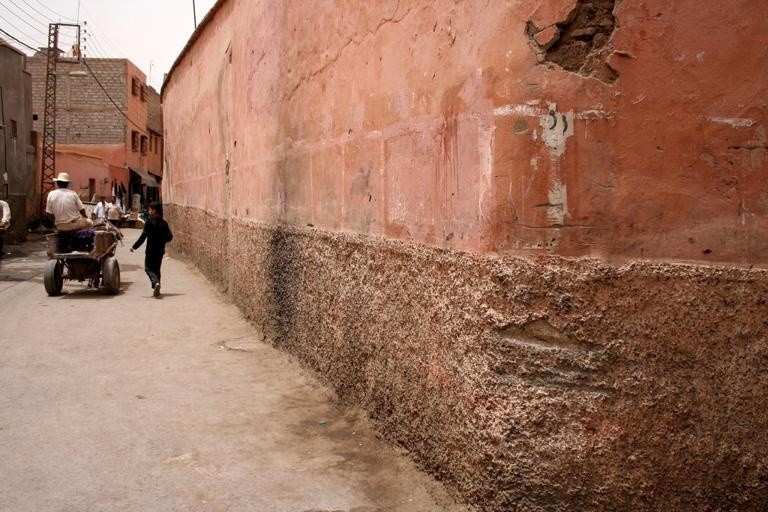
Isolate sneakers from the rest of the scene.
[152,282,162,297]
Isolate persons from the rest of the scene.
[92,196,110,219]
[105,196,123,230]
[0,200,13,262]
[44,171,95,231]
[129,200,174,299]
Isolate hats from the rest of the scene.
[52,171,72,184]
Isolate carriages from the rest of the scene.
[44,211,121,295]
